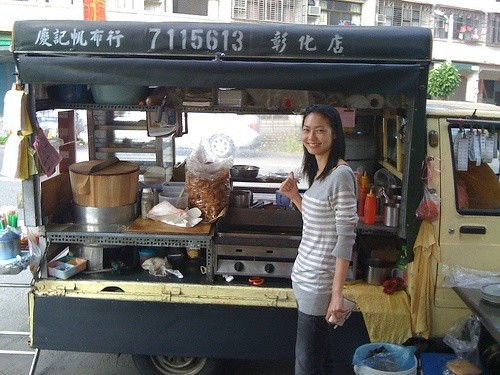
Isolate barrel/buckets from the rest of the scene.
[68,158,140,207]
[230,190,251,208]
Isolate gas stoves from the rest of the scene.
[212,202,302,279]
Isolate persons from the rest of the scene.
[280,104,359,375]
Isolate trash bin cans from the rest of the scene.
[354,343,417,375]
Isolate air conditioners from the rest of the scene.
[307,6,321,18]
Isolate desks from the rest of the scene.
[462,160,500,209]
[452,287,500,347]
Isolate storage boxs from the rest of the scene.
[47,256,88,280]
[419,352,488,375]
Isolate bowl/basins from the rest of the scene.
[162,182,185,192]
[89,84,149,105]
[362,257,393,285]
[158,192,188,209]
[230,165,260,178]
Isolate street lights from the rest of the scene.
[433,8,455,101]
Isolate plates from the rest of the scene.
[480,282,500,304]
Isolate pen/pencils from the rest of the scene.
[334,324,338,329]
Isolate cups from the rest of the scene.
[139,249,154,266]
[0,236,21,260]
[384,203,400,227]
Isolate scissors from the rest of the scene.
[249,277,264,285]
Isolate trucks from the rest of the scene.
[12,15,500,374]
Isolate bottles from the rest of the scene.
[356,169,376,225]
[141,188,158,220]
[396,245,407,278]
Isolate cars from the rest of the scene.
[78,111,263,159]
